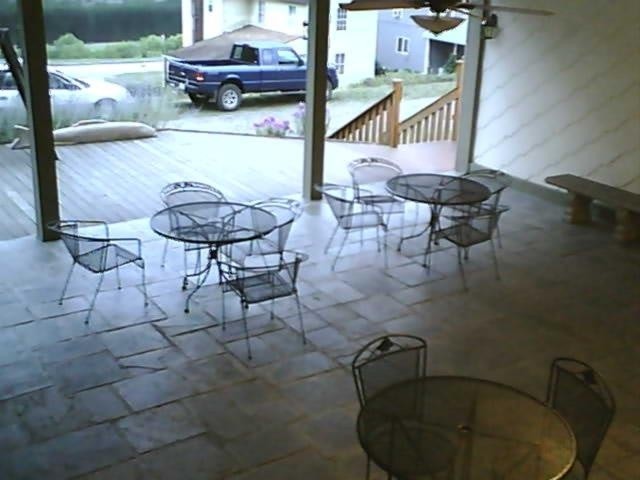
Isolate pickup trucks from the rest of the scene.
[164,41,339,111]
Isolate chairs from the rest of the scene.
[425,204,512,291]
[217,250,310,360]
[348,158,408,245]
[353,335,456,480]
[434,169,513,260]
[222,196,304,309]
[313,183,390,270]
[160,181,236,285]
[47,221,147,326]
[546,356,617,480]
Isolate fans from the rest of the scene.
[338,0,554,35]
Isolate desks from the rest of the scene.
[357,376,577,480]
[386,174,492,268]
[150,201,277,315]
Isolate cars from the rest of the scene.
[0,63,136,143]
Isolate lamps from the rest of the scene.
[479,14,502,39]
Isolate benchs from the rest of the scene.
[545,173,640,245]
